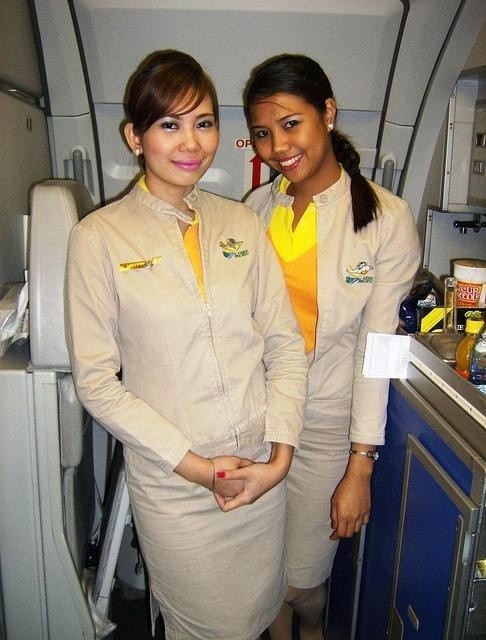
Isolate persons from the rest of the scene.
[61,48,309,639]
[230,52,425,638]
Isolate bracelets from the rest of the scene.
[347,447,381,462]
[208,457,217,492]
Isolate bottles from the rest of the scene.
[454,316,486,385]
[429,276,465,361]
